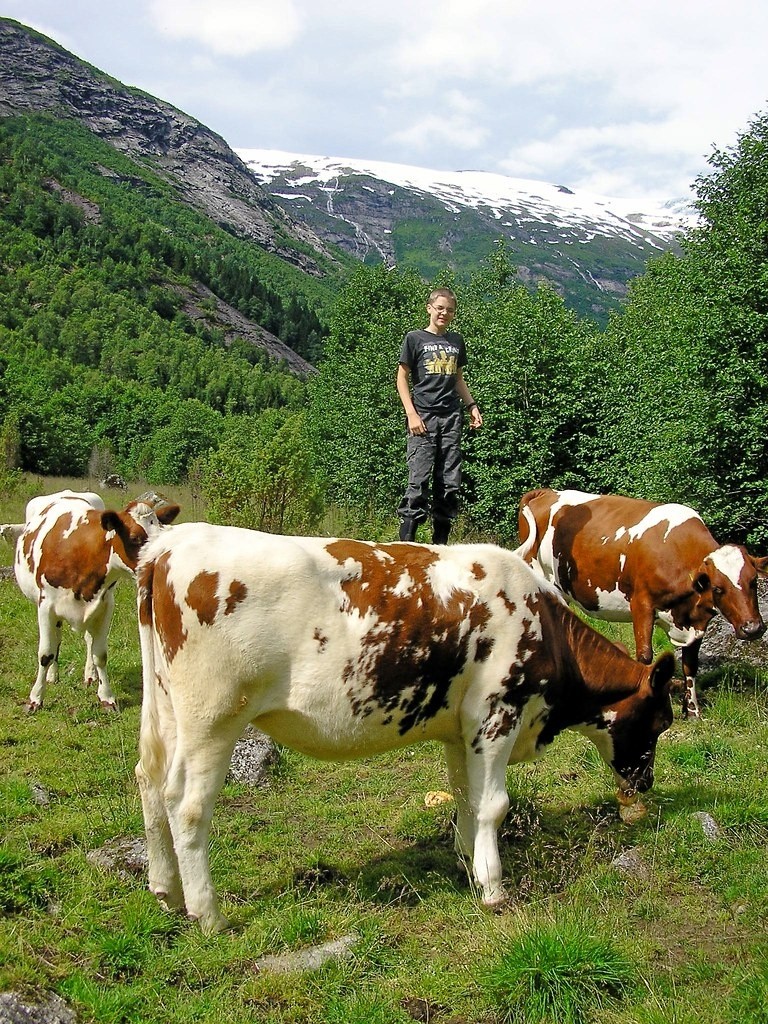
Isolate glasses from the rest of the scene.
[431,304,456,315]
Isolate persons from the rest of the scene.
[396,289,483,545]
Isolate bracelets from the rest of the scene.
[467,402,476,411]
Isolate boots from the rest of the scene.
[432,520,450,545]
[399,517,418,542]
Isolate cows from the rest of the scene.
[15,491,180,719]
[134,518,676,941]
[0,523,26,547]
[518,488,767,720]
